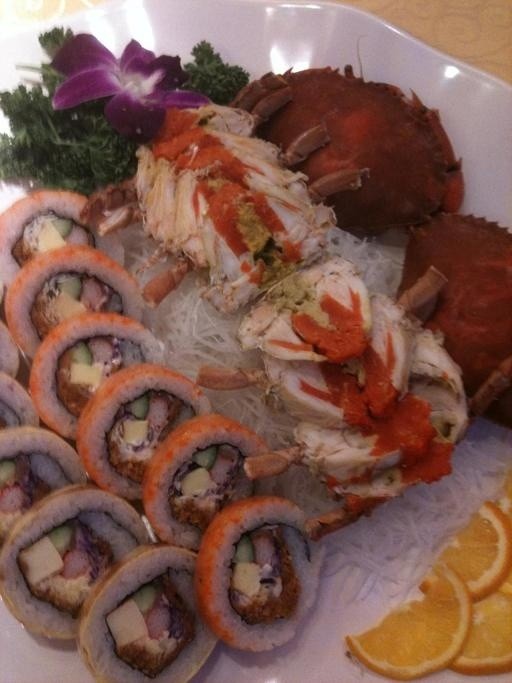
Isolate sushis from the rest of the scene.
[0,189,321,683]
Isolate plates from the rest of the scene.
[0,2,512,683]
